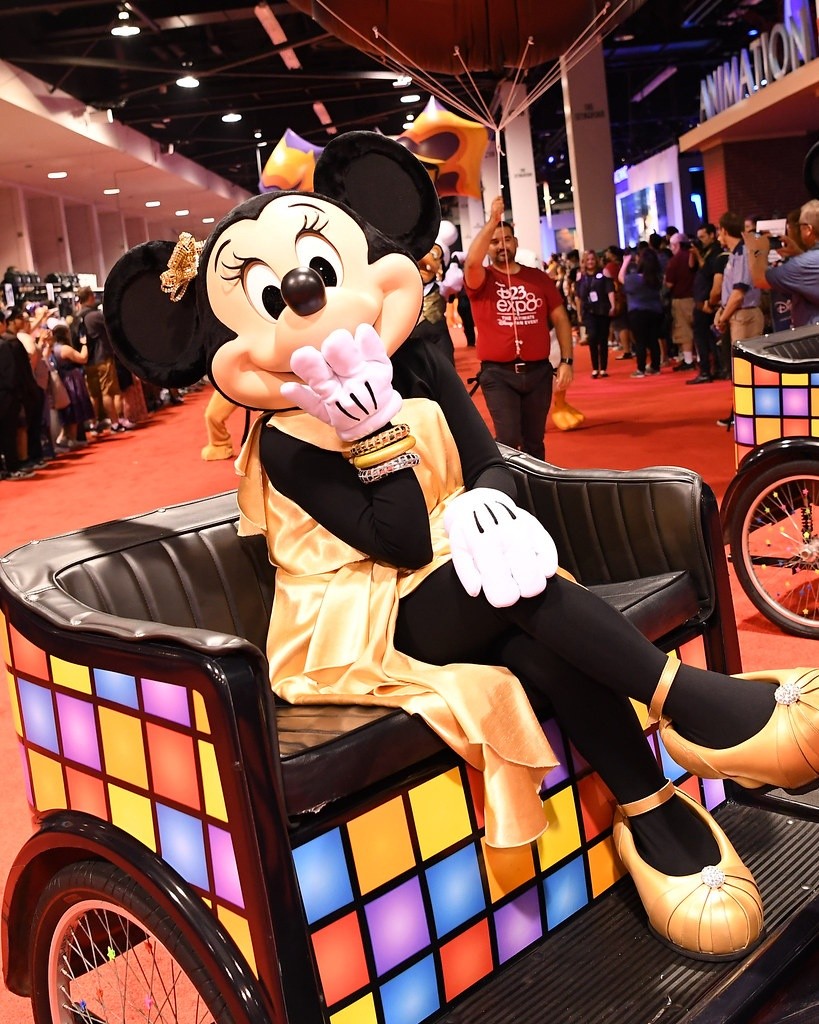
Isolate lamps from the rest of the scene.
[161,142,174,155]
[111,7,140,36]
[84,108,114,127]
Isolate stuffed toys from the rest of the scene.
[415,217,464,368]
[104,130,819,959]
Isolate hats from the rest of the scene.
[6,307,22,320]
[0,307,12,322]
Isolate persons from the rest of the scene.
[460,196,574,462]
[449,264,476,347]
[543,198,819,427]
[0,286,205,478]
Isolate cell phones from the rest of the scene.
[767,236,782,249]
[80,331,83,338]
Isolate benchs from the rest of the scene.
[732,324,819,474]
[0,442,741,1023]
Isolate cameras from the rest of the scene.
[679,239,703,251]
[630,256,636,261]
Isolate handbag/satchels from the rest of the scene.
[49,370,71,409]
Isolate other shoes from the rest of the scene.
[645,365,660,375]
[630,369,644,378]
[6,468,36,480]
[111,423,127,433]
[592,370,598,378]
[616,352,632,360]
[673,358,694,371]
[68,439,87,448]
[31,459,47,468]
[119,418,136,428]
[90,422,102,435]
[600,370,608,377]
[56,436,73,448]
[717,416,734,426]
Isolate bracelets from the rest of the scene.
[560,358,573,365]
[349,423,420,484]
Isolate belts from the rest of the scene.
[486,360,548,373]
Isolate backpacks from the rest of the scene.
[69,309,95,366]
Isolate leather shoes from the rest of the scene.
[686,371,713,384]
